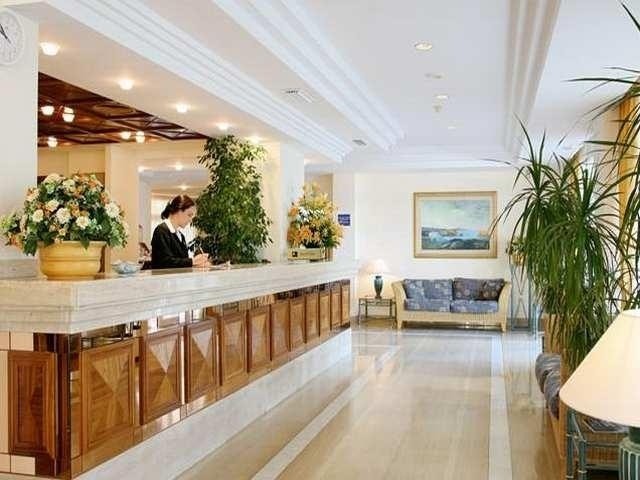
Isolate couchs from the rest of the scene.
[391,276,512,333]
[533,312,628,480]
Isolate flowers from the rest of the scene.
[286,182,343,247]
[1,171,129,257]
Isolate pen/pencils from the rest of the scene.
[199,247,204,254]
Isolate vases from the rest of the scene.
[288,248,325,260]
[374,275,384,299]
[36,238,107,280]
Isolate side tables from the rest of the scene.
[357,296,396,329]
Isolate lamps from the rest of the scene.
[41,105,145,148]
[558,307,640,480]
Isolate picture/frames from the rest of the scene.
[413,191,498,260]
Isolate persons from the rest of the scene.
[139,242,149,258]
[151,195,211,270]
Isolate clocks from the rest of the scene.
[0,10,25,67]
